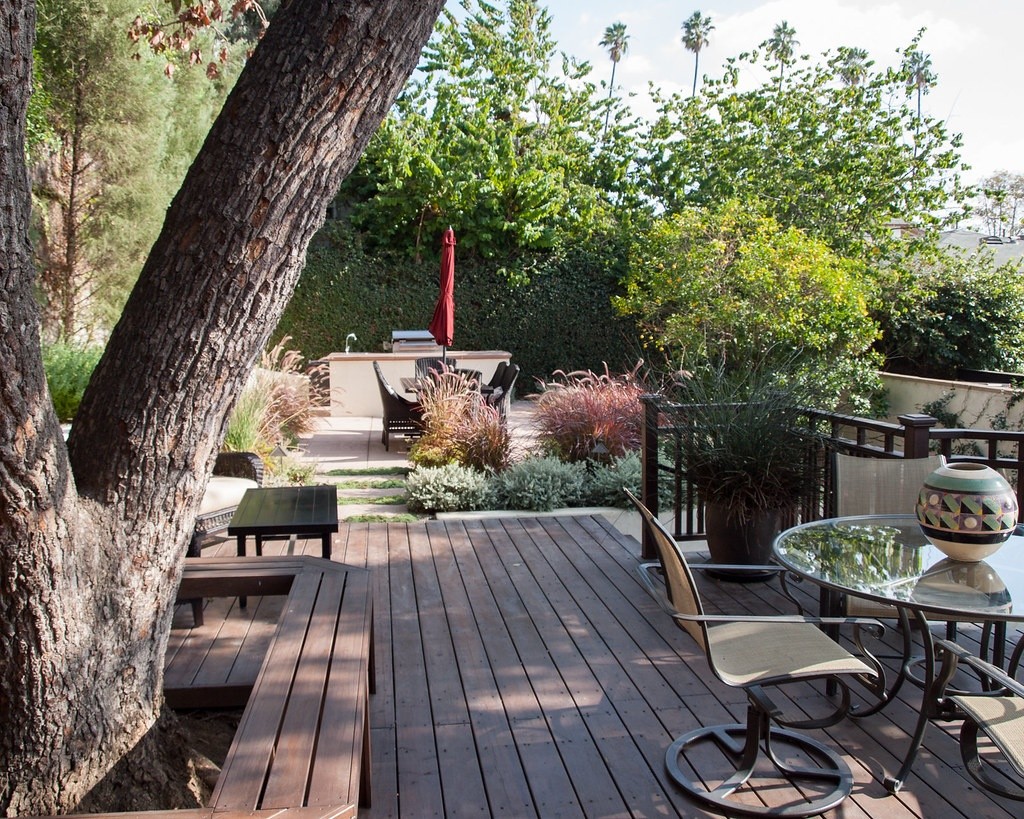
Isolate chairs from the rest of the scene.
[414,357,456,378]
[373,360,427,452]
[59,421,263,626]
[488,361,509,388]
[487,361,520,416]
[432,368,482,394]
[934,633,1024,802]
[622,485,888,818]
[819,450,1007,696]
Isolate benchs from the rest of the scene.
[176,555,370,627]
[208,571,380,808]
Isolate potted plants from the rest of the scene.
[624,321,849,572]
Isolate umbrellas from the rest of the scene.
[428,225,456,364]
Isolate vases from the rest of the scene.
[914,462,1019,562]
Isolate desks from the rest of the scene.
[226,485,339,608]
[319,350,513,417]
[771,513,1024,793]
[400,377,494,393]
